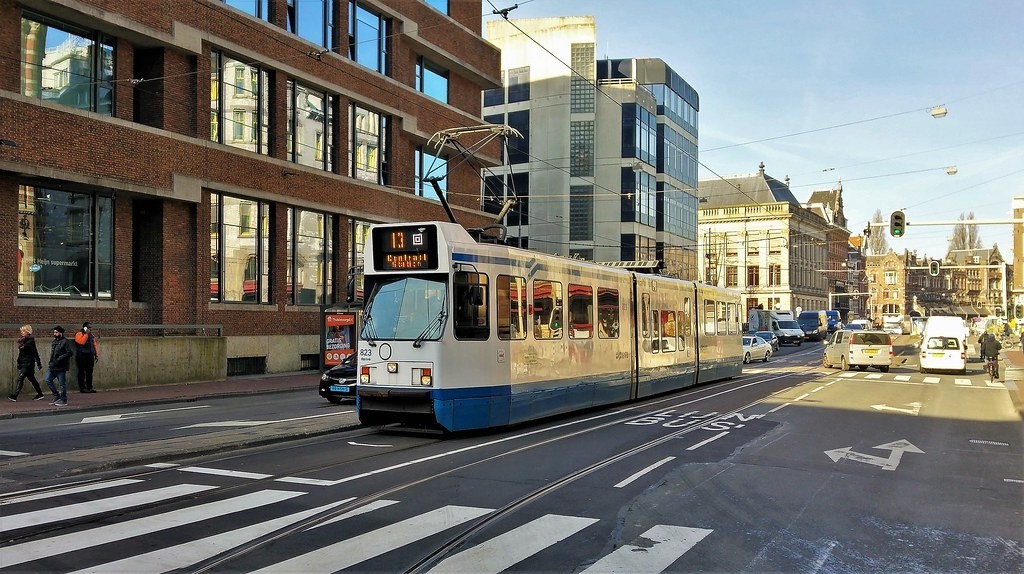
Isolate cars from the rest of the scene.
[842,323,862,330]
[319,352,357,405]
[743,330,781,364]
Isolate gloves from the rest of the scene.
[38,366,42,370]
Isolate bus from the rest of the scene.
[354,125,744,435]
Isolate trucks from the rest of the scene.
[747,308,806,346]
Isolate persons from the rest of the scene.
[75,323,101,394]
[8,325,44,401]
[976,322,1024,379]
[47,327,76,407]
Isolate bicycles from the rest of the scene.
[985,357,999,382]
[979,341,986,359]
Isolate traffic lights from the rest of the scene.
[929,261,940,276]
[889,210,905,238]
[849,235,861,247]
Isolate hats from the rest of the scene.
[53,326,64,333]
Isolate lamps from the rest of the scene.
[631,162,643,173]
[932,106,948,118]
[699,197,708,204]
[947,167,959,175]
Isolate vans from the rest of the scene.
[822,329,893,372]
[851,319,872,330]
[883,312,904,335]
[797,310,842,341]
[919,315,969,374]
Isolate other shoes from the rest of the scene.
[48,396,60,405]
[89,389,96,392]
[33,394,45,400]
[80,389,89,393]
[8,395,17,402]
[55,399,67,406]
